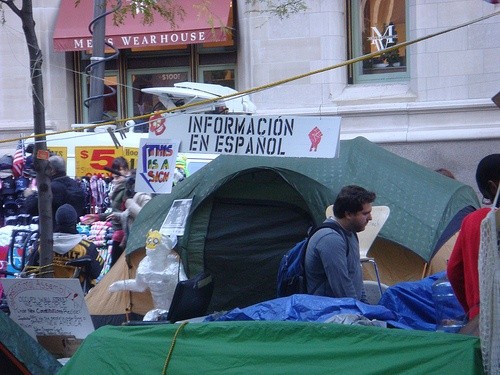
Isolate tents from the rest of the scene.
[52,136,482,375]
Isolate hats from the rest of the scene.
[0,153,40,225]
[56,204,76,225]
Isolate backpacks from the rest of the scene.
[277,222,349,297]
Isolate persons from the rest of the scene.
[108,232,188,322]
[447,154,500,321]
[0,143,152,298]
[304,185,376,302]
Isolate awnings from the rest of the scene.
[52,0,236,52]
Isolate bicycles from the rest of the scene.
[0,256,94,313]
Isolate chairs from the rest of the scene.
[122,271,214,326]
[326,205,391,297]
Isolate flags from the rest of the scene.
[11,133,27,179]
